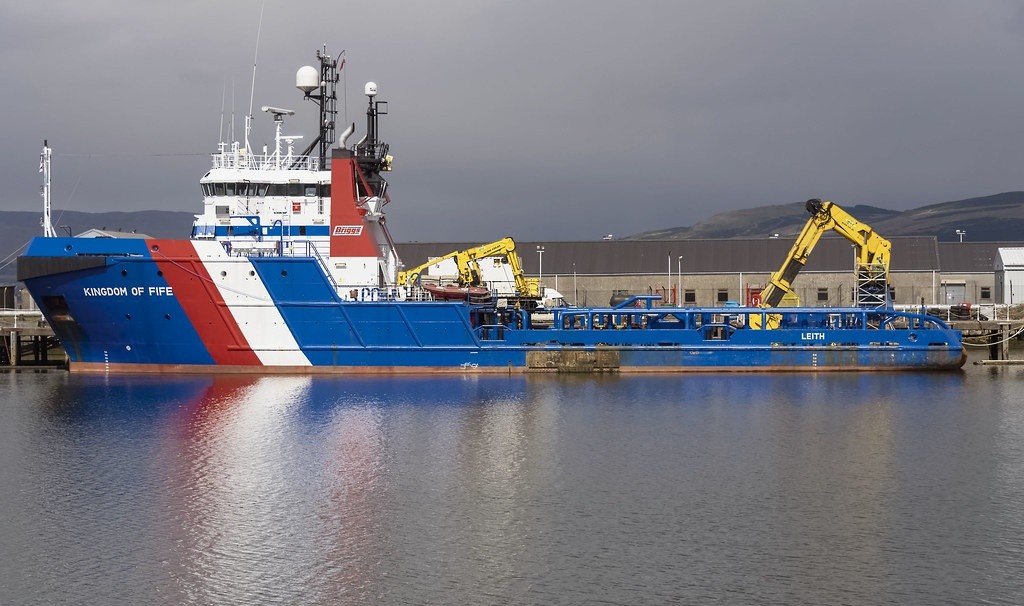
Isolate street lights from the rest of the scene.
[678,256,684,307]
[535,244,545,298]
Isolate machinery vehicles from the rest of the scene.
[398,236,540,309]
[745,197,892,331]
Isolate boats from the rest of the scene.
[15,2,967,376]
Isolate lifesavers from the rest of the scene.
[930,305,988,321]
